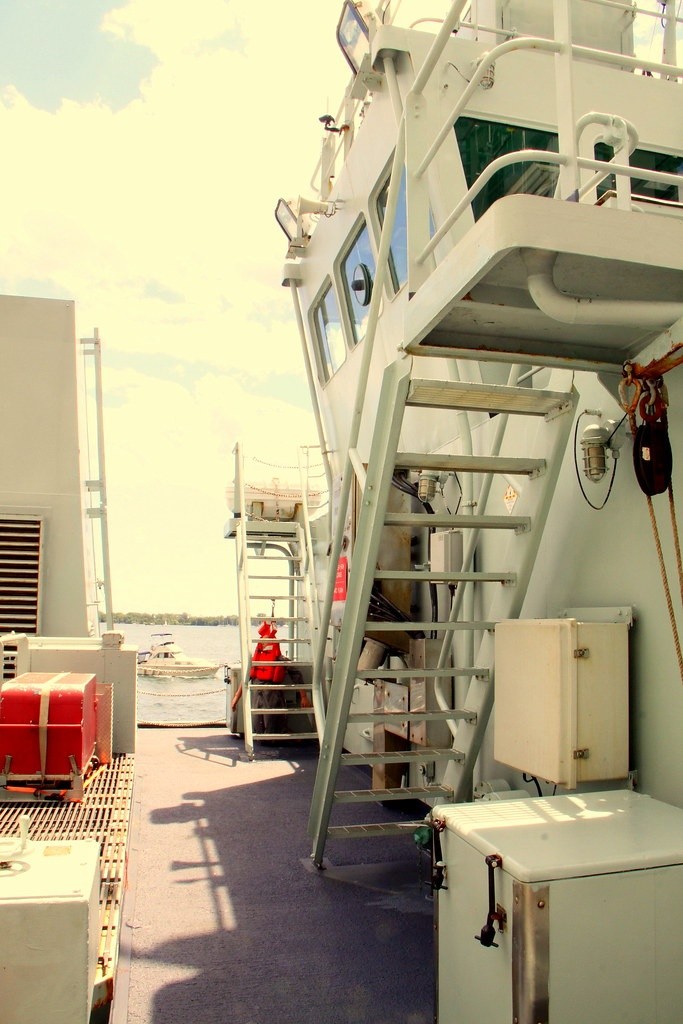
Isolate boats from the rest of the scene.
[138,632,224,679]
